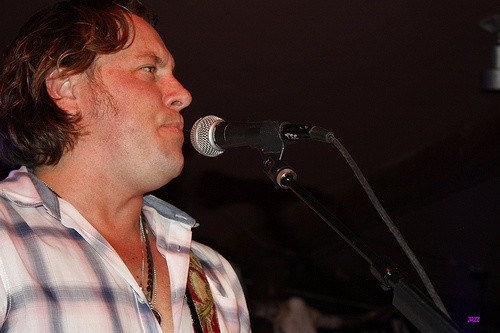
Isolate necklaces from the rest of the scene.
[140,212,162,326]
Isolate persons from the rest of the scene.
[0,0,252,333]
[249,182,412,333]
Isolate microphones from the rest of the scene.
[190,115,333,157]
